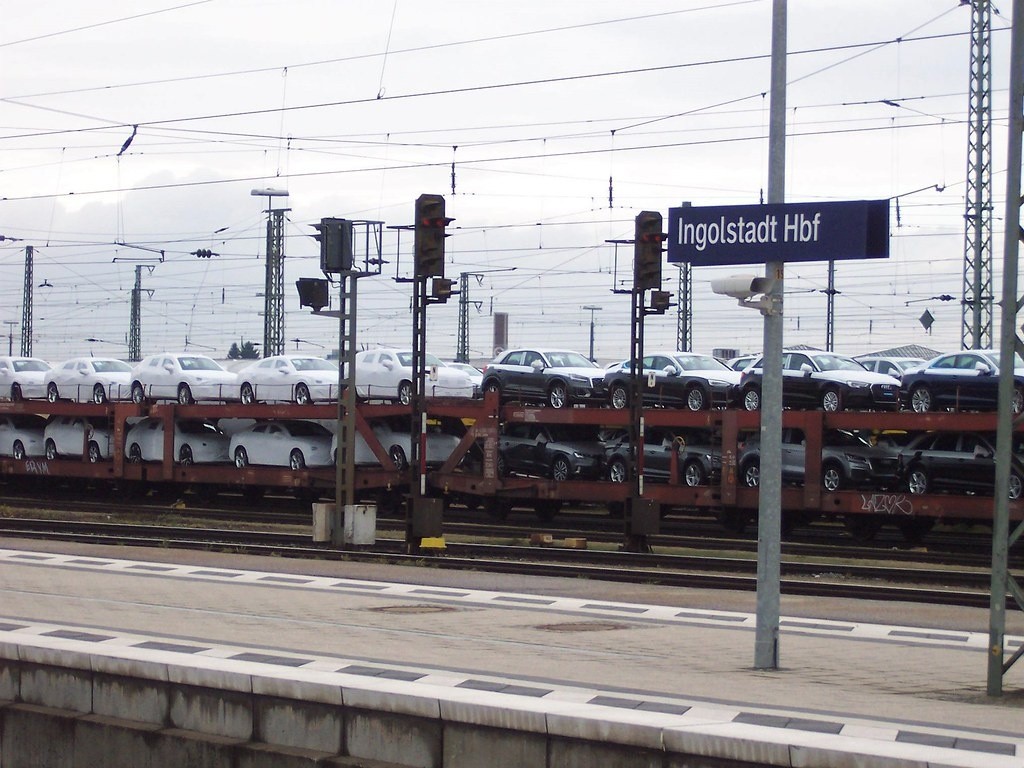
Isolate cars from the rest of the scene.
[1,351,1024,414]
[0,414,1024,501]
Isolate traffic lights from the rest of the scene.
[413,193,448,279]
[634,211,667,293]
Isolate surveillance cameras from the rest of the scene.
[711,275,773,299]
